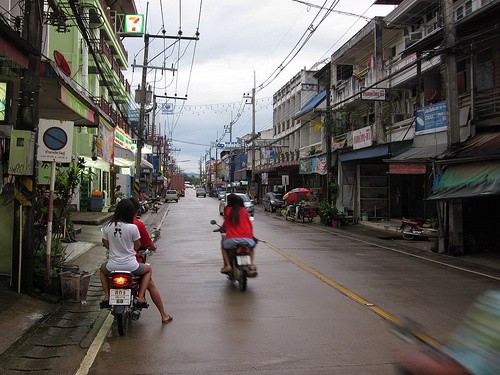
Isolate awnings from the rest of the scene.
[294,90,326,121]
[382,143,447,175]
[339,116,417,161]
[423,160,500,201]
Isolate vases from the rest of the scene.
[60,270,91,303]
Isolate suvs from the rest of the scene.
[164,189,179,203]
[262,192,286,213]
[218,192,254,217]
[196,188,207,198]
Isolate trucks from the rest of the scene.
[170,175,185,196]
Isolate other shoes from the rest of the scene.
[162,314,173,323]
[99,300,108,306]
[221,268,232,272]
[137,298,147,305]
[248,264,256,271]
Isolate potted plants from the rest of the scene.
[317,202,332,225]
[33,164,66,294]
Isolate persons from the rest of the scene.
[215,194,257,273]
[129,198,173,325]
[100,199,152,303]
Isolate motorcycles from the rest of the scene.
[105,229,160,336]
[211,216,257,292]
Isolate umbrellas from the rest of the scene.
[283,188,309,202]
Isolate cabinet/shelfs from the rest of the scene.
[356,164,391,221]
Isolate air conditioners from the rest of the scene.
[391,113,408,124]
